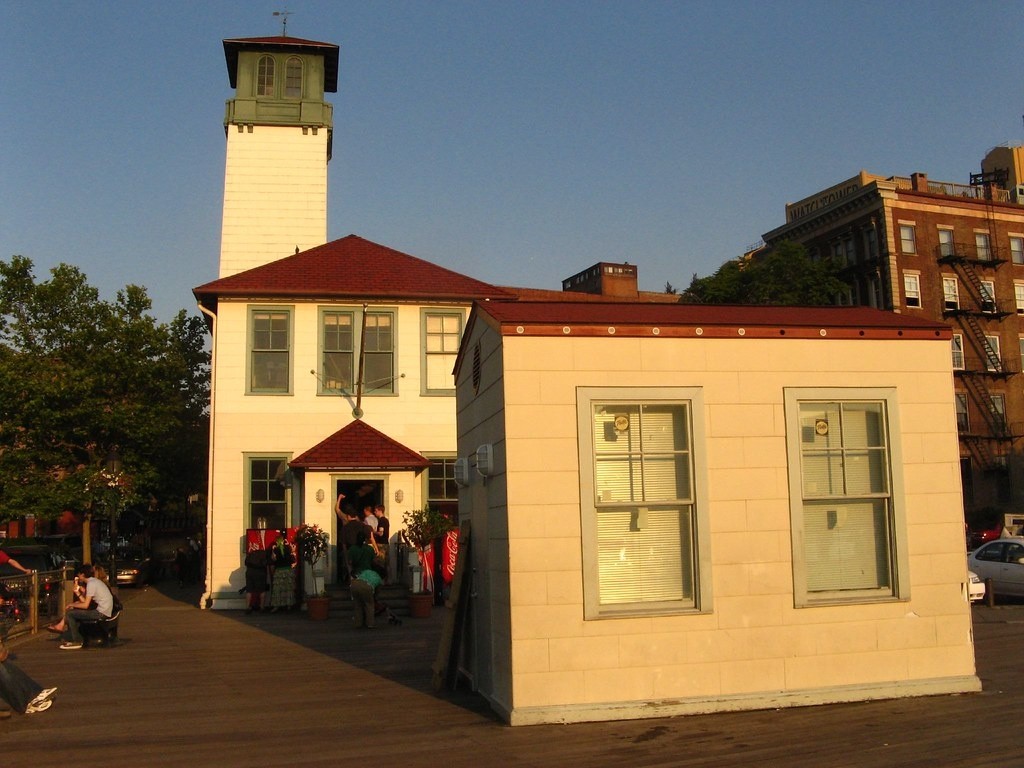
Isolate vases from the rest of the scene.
[306,597,333,621]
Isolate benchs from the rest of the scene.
[78,610,120,649]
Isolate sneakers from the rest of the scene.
[25,686,59,715]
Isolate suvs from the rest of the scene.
[1,543,82,616]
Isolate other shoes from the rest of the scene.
[59,640,84,649]
[367,626,380,629]
[244,607,253,615]
[260,609,264,613]
[355,626,363,630]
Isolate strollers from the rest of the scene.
[349,574,403,626]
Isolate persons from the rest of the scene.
[173,539,204,589]
[0,639,58,716]
[335,494,392,587]
[0,535,32,614]
[244,539,297,614]
[351,568,386,629]
[48,562,122,651]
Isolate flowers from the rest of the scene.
[292,525,329,599]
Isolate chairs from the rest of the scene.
[1010,551,1024,563]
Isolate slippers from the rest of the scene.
[46,623,64,636]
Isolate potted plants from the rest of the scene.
[402,504,455,618]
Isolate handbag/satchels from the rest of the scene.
[112,595,123,616]
[350,579,373,599]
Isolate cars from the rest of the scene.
[966,538,1024,598]
[98,556,143,587]
[966,568,986,604]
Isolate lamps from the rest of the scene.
[476,443,494,479]
[317,489,324,502]
[396,489,403,503]
[453,457,470,487]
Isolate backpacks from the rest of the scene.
[245,551,267,567]
